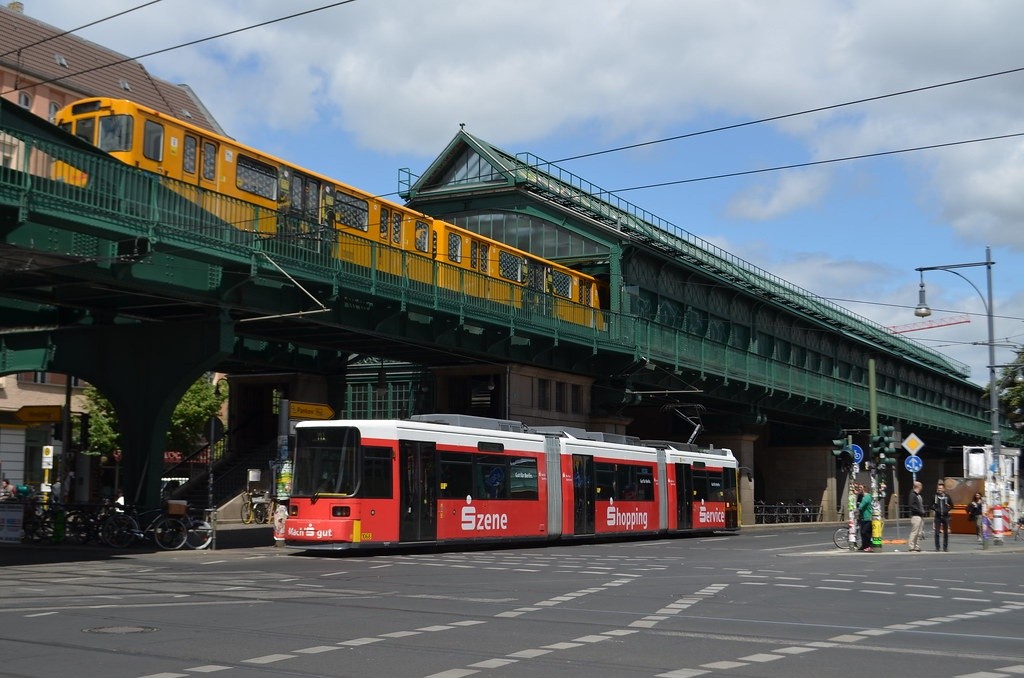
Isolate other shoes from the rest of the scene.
[864,547,873,551]
[935,549,939,552]
[944,549,949,552]
[858,547,863,550]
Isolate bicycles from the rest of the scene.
[834,524,863,549]
[240,491,267,524]
[754,499,820,523]
[17,497,213,551]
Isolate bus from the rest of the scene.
[53,94,606,331]
[271,413,740,551]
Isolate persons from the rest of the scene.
[929,483,953,552]
[967,491,984,542]
[907,481,926,551]
[114,490,124,515]
[852,481,874,552]
[51,476,61,505]
[0,478,16,502]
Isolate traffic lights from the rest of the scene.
[868,434,885,460]
[832,438,847,459]
[880,425,896,466]
[840,450,854,465]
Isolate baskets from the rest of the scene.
[168,500,187,514]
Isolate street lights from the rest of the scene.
[914,261,1024,546]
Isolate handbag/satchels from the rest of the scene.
[968,514,976,521]
